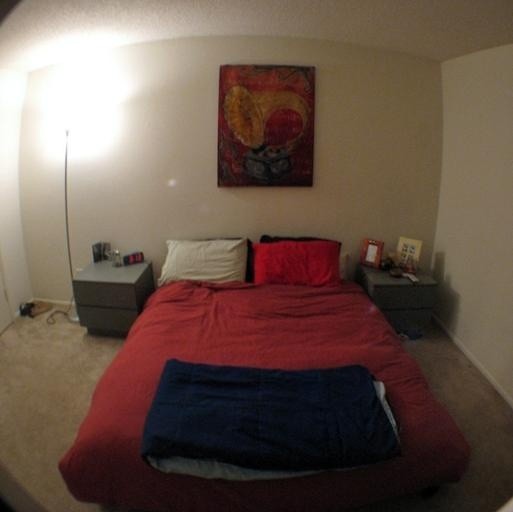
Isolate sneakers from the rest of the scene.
[19,301,53,316]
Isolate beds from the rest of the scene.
[59,278,469,511]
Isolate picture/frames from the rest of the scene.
[358,238,383,268]
[392,237,424,273]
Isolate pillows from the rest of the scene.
[157,233,342,288]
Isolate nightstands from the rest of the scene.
[71,257,153,338]
[356,261,439,341]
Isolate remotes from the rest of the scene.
[407,272,419,283]
